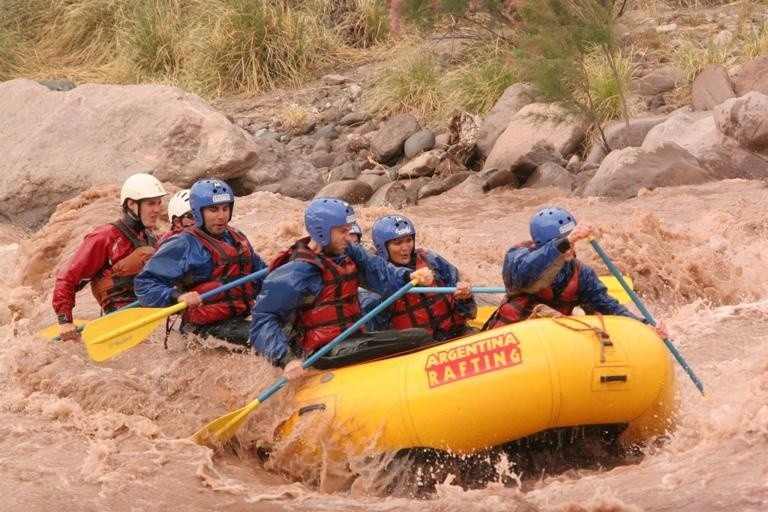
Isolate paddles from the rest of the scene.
[188,272,424,450]
[586,232,708,398]
[403,275,633,305]
[82,267,270,361]
[54,300,141,344]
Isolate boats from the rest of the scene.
[34,305,670,477]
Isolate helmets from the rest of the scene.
[372,215,416,267]
[305,198,356,252]
[120,173,168,229]
[189,178,234,236]
[168,189,190,230]
[349,223,362,243]
[530,207,577,249]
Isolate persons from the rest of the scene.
[168,189,196,230]
[358,215,477,340]
[52,174,169,342]
[348,222,363,242]
[479,207,669,340]
[249,198,433,382]
[135,179,269,345]
